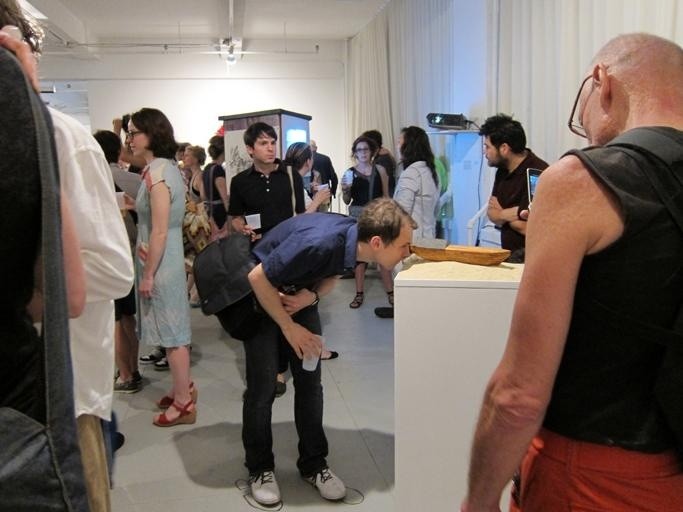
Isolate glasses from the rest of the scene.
[128,129,141,139]
[567,74,593,138]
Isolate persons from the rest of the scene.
[478,113,550,264]
[1,2,441,511]
[461,32,683,512]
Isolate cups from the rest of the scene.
[315,182,332,208]
[111,190,129,221]
[300,331,325,373]
[245,212,264,241]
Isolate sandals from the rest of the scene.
[350,292,363,307]
[388,292,394,304]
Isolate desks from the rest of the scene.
[394,253,525,512]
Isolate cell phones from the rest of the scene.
[526,168,543,207]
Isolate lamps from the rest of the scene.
[225,46,237,65]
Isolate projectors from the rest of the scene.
[426,112,468,129]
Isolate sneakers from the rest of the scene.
[115,347,346,506]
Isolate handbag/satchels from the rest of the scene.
[347,204,366,217]
[192,234,262,334]
[1,404,90,512]
[209,216,230,240]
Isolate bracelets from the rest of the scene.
[518,214,527,221]
